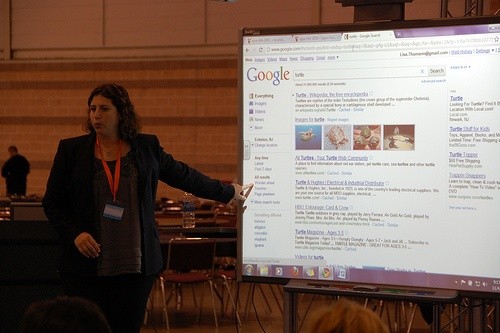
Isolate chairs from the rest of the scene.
[144,233,300,330]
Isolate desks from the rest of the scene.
[157,237,237,271]
[182,228,239,237]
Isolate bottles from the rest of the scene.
[181,190,195,229]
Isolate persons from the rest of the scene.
[20,297,112,333]
[42,83,246,333]
[301,301,390,333]
[0,145,30,196]
